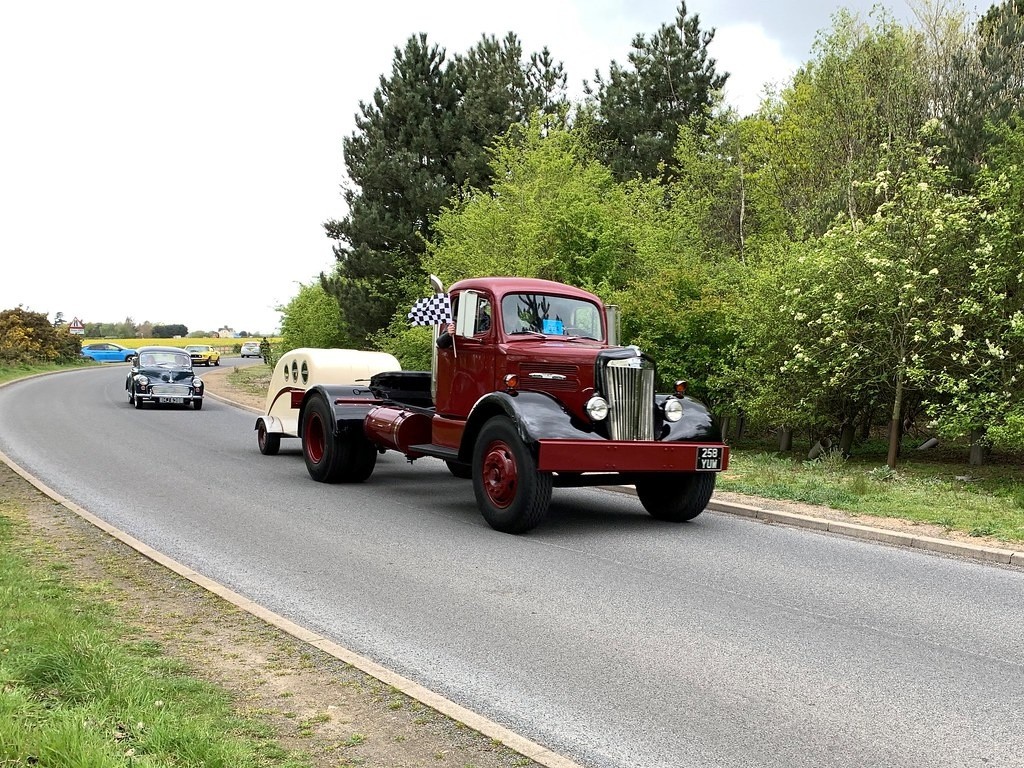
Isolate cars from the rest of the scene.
[125,347,205,410]
[240,342,261,358]
[80,342,136,362]
[184,344,220,366]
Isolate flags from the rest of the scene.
[407,293,452,327]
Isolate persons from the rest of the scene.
[436,309,490,349]
[175,356,186,365]
[143,356,155,365]
[260,337,271,364]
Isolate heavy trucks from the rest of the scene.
[254,276,729,539]
